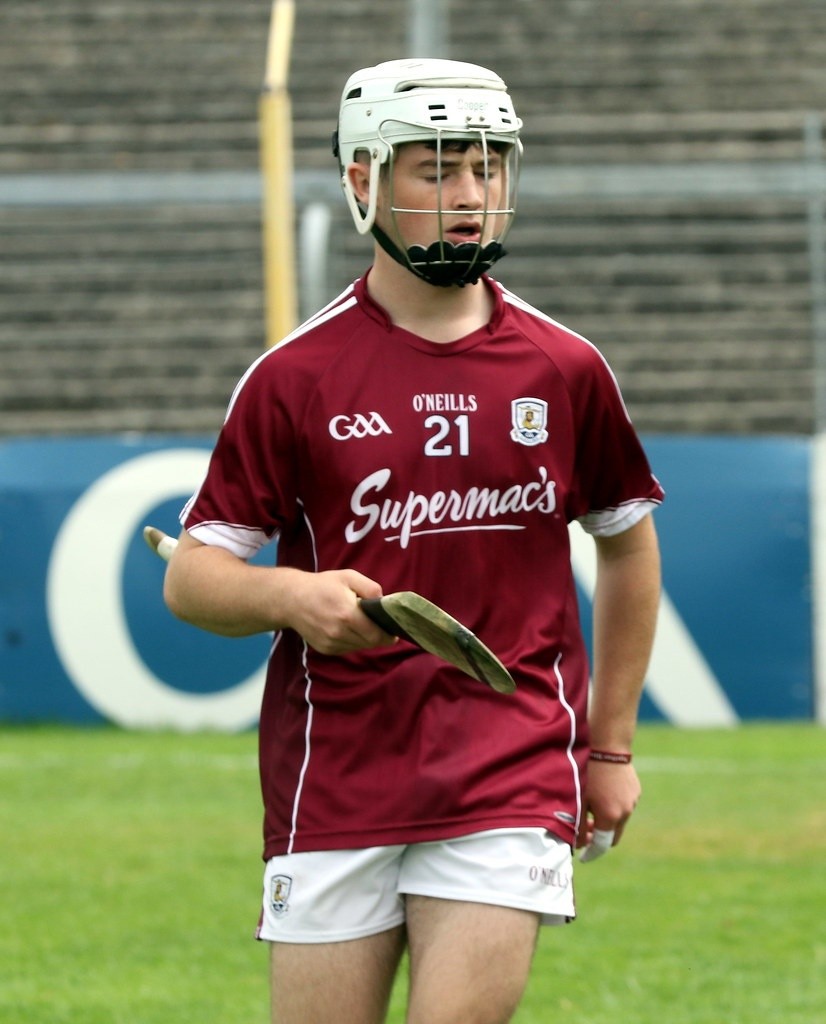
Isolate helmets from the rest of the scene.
[333,58,525,286]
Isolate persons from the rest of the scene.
[164,56,664,1024]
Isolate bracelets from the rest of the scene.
[589,749,632,764]
